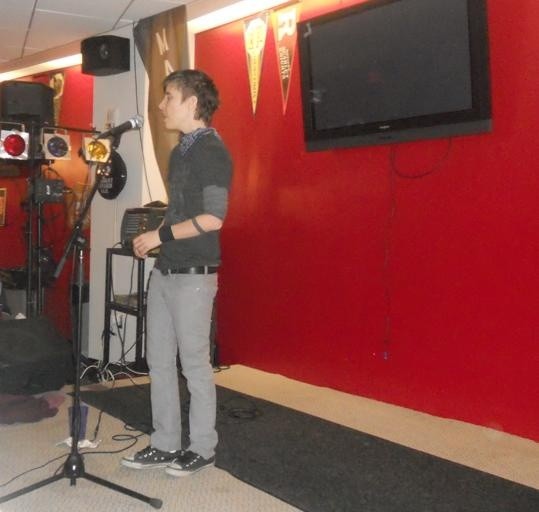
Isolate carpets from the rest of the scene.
[66,384,539,511]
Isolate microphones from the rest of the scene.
[98,114,145,140]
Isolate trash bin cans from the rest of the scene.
[3,266,45,318]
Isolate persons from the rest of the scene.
[121,70,233,476]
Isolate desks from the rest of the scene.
[101,249,224,376]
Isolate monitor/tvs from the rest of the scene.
[295,0,495,152]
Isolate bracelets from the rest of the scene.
[159,225,175,242]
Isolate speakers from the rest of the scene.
[0,316,74,395]
[79,34,132,77]
[0,80,55,166]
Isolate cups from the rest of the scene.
[68,406,89,442]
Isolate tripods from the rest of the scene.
[0,142,164,510]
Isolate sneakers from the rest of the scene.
[121,445,216,477]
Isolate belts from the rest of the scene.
[162,266,217,274]
